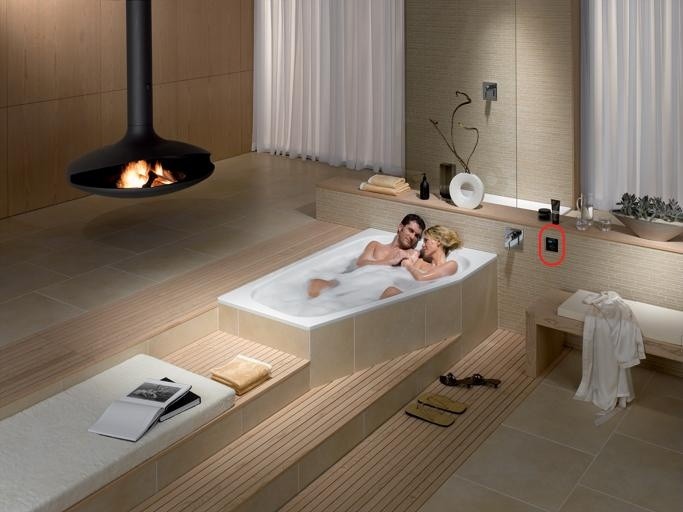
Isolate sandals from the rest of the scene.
[440,372,501,388]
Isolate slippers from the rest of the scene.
[405,393,467,427]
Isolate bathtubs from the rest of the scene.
[216,226,499,330]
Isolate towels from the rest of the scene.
[359,174,411,195]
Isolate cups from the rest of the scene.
[576,192,593,228]
[576,215,590,232]
[599,219,612,231]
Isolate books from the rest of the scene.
[87,377,202,443]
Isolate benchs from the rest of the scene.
[524,288,683,369]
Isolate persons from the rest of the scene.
[304,213,464,299]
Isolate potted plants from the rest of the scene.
[609,192,683,241]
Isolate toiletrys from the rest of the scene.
[537,197,560,225]
